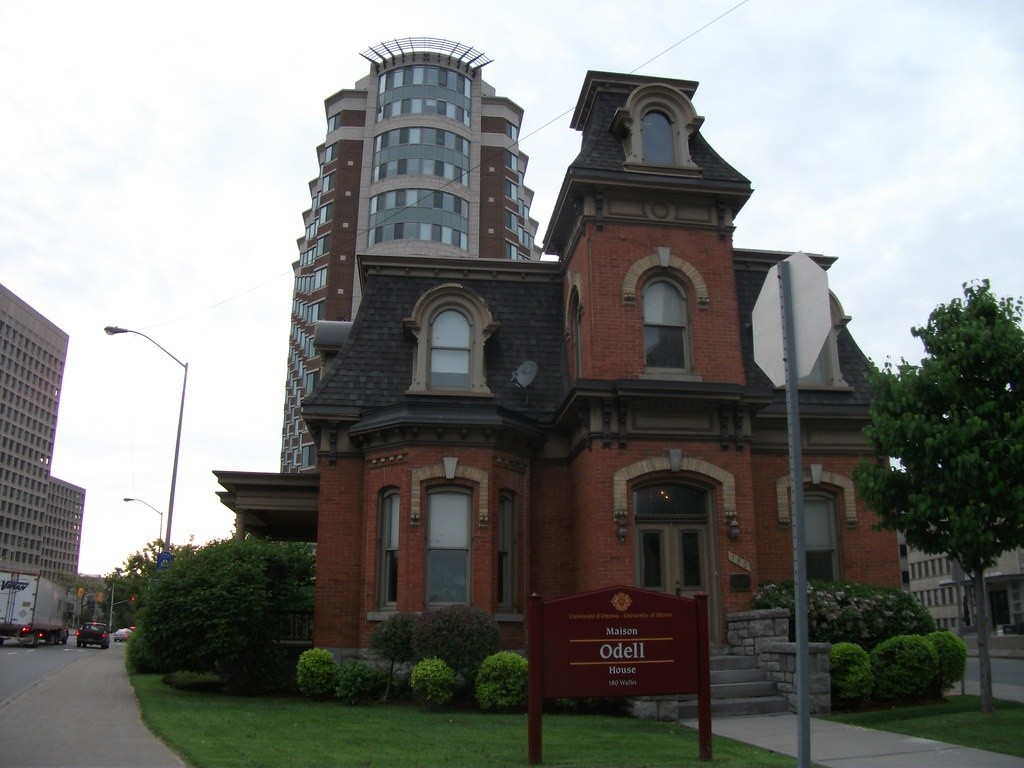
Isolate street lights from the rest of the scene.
[122,497,164,555]
[103,325,189,548]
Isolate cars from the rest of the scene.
[113,628,133,642]
[76,622,112,649]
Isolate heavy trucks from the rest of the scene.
[0,569,75,648]
